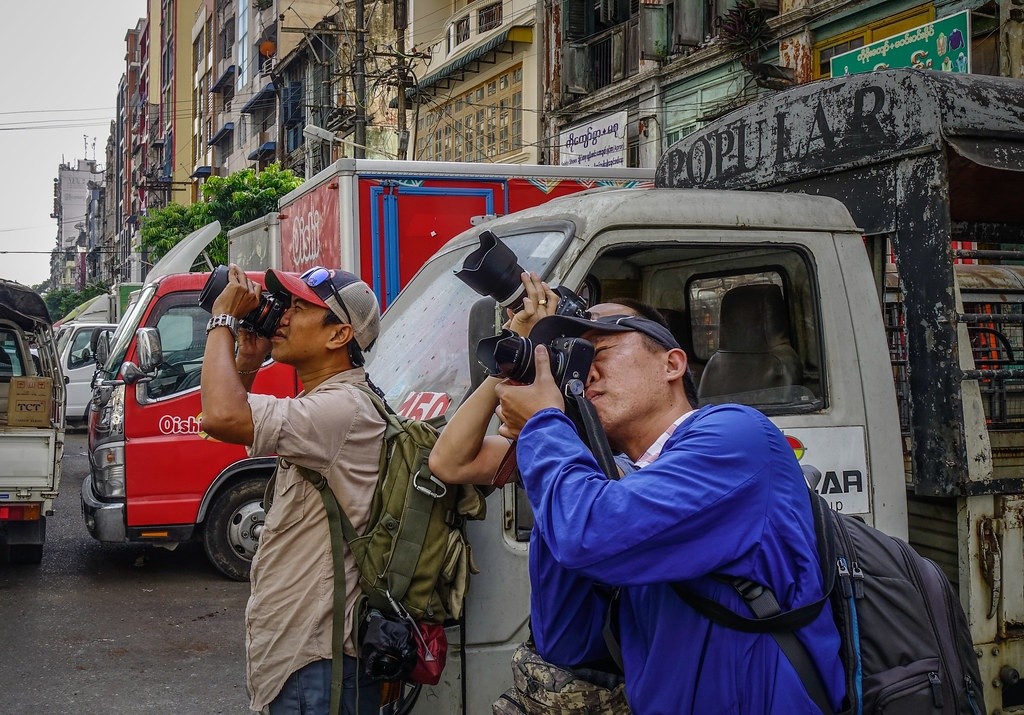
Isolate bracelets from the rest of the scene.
[236,368,260,375]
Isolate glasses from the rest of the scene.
[299,266,351,326]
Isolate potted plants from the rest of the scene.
[710,0,775,70]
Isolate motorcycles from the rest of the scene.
[0,277,72,567]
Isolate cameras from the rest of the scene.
[451,230,592,319]
[476,327,596,405]
[197,265,290,340]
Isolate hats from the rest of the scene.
[264,267,381,350]
[529,315,691,379]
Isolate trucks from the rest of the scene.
[358,67,1024,715]
[49,156,655,584]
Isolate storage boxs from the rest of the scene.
[6,376,53,427]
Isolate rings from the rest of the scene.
[538,299,547,306]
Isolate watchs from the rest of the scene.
[205,314,239,341]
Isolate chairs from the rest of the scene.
[650,309,704,385]
[697,284,803,399]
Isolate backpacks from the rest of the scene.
[292,382,486,620]
[646,411,986,715]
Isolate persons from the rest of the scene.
[428,270,669,715]
[201,263,392,715]
[496,315,849,715]
